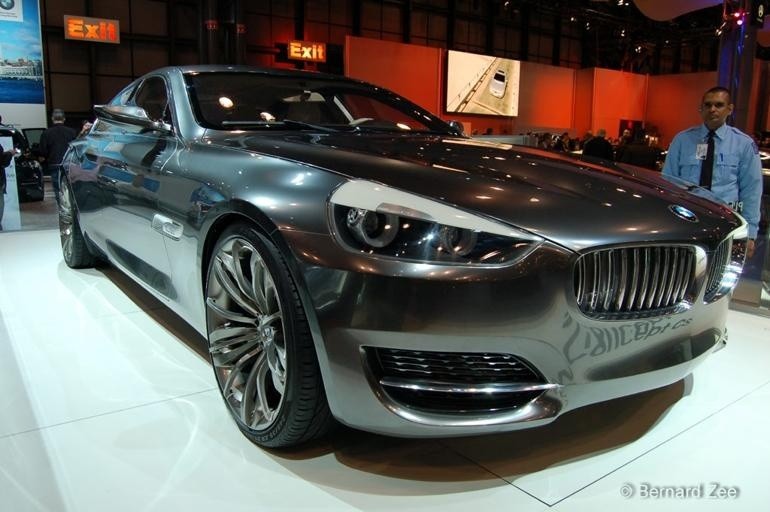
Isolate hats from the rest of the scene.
[51,62,756,465]
[487,67,508,100]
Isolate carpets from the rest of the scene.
[700,130,716,191]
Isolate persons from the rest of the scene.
[75,119,92,140]
[38,107,78,202]
[661,87,764,257]
[471,127,660,170]
[1,145,16,232]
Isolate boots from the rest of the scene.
[0,124,47,203]
